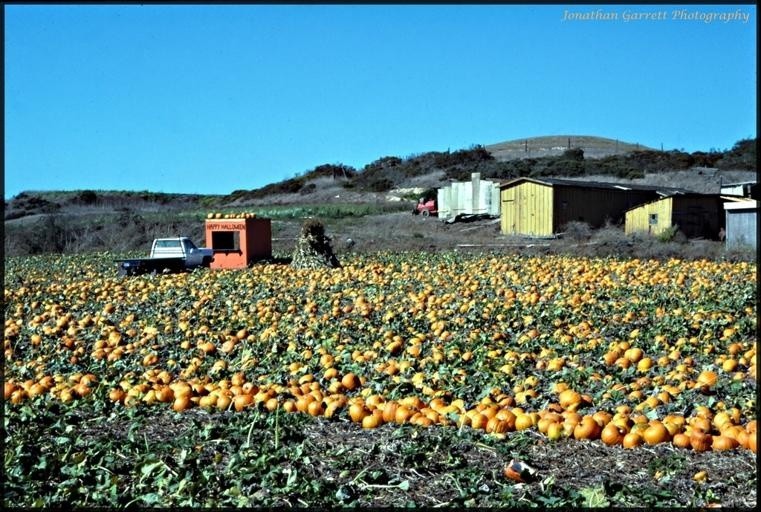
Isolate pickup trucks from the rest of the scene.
[113,237,214,276]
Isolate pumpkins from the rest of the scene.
[0,212,757,481]
